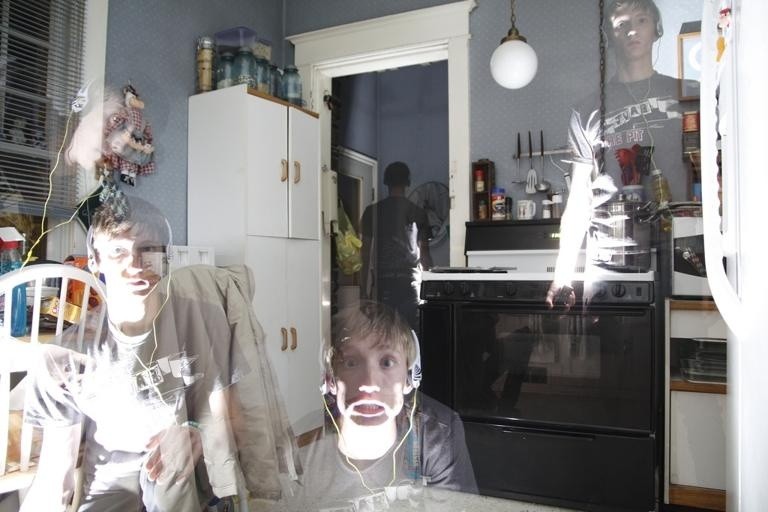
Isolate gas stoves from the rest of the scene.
[417,264,656,305]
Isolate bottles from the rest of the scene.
[474,170,512,221]
[0,240,28,337]
[540,194,563,219]
[649,169,672,205]
[195,36,302,107]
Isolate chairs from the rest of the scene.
[1,262,107,512]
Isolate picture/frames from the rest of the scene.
[678,31,701,101]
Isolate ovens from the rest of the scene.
[417,303,661,511]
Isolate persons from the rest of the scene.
[0,1,726,510]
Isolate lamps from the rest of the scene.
[490,1,538,90]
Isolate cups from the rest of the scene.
[516,199,536,220]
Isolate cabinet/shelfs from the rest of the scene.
[187,83,318,238]
[663,302,726,509]
[189,240,323,454]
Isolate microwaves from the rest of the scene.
[670,214,714,299]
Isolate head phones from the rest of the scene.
[601,0,663,47]
[85,199,175,279]
[71,76,97,113]
[384,161,411,186]
[316,299,422,397]
